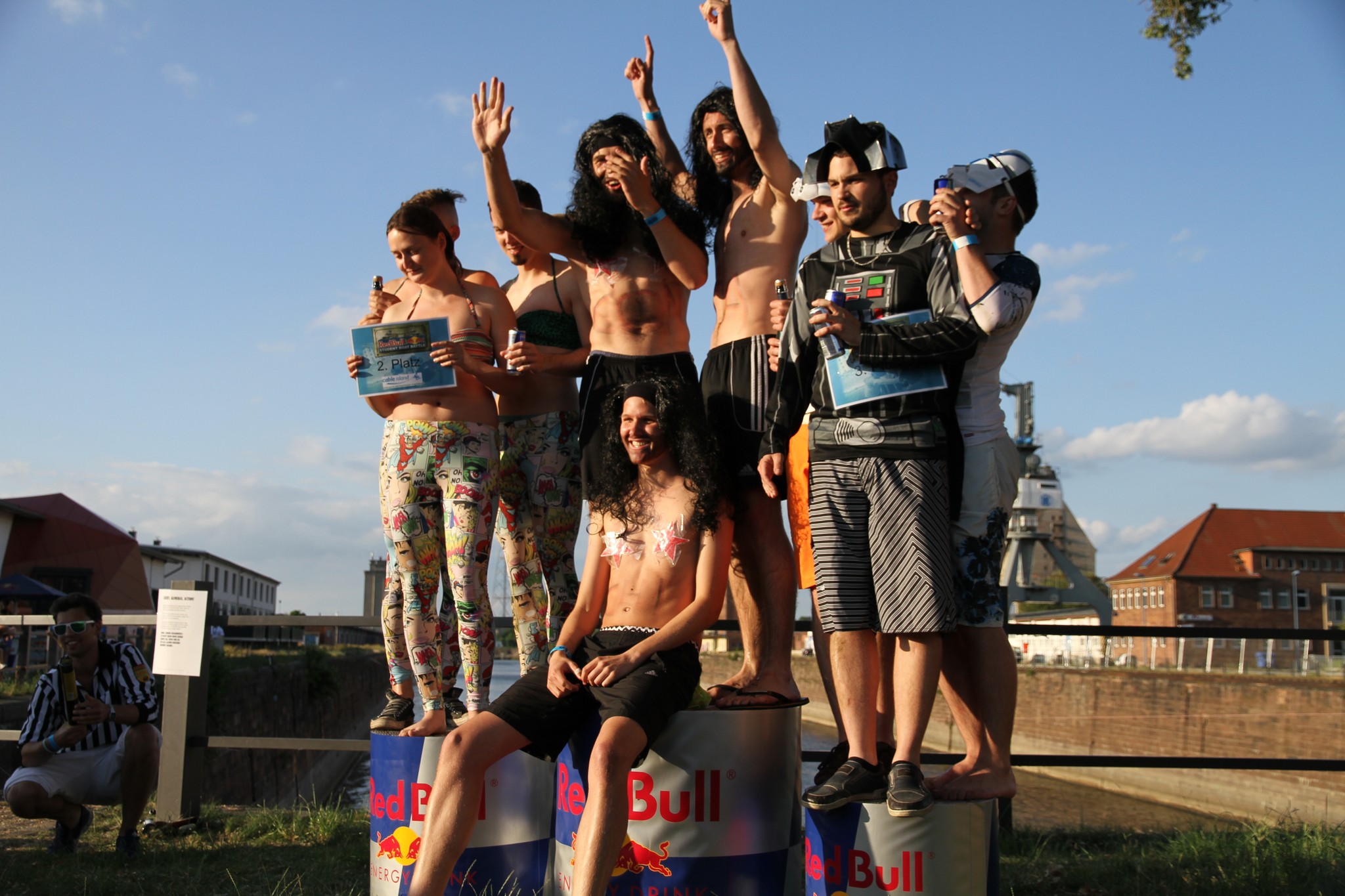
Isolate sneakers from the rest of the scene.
[370,688,415,730]
[47,805,93,854]
[116,829,140,856]
[813,740,850,786]
[442,687,469,728]
[876,741,896,774]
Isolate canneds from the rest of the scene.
[807,306,846,361]
[824,288,847,306]
[506,326,526,376]
[932,175,955,235]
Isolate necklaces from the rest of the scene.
[846,218,903,266]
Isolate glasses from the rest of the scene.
[52,620,95,636]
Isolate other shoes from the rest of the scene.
[886,764,933,817]
[800,761,887,811]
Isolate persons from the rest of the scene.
[4,595,164,859]
[753,119,1043,819]
[472,0,812,710]
[407,376,735,896]
[344,178,590,737]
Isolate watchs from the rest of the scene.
[108,703,116,722]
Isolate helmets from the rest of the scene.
[790,176,831,203]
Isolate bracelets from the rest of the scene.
[951,234,979,252]
[639,208,666,227]
[541,643,574,657]
[641,108,663,120]
[42,734,58,757]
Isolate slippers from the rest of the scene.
[707,684,740,705]
[715,687,810,710]
